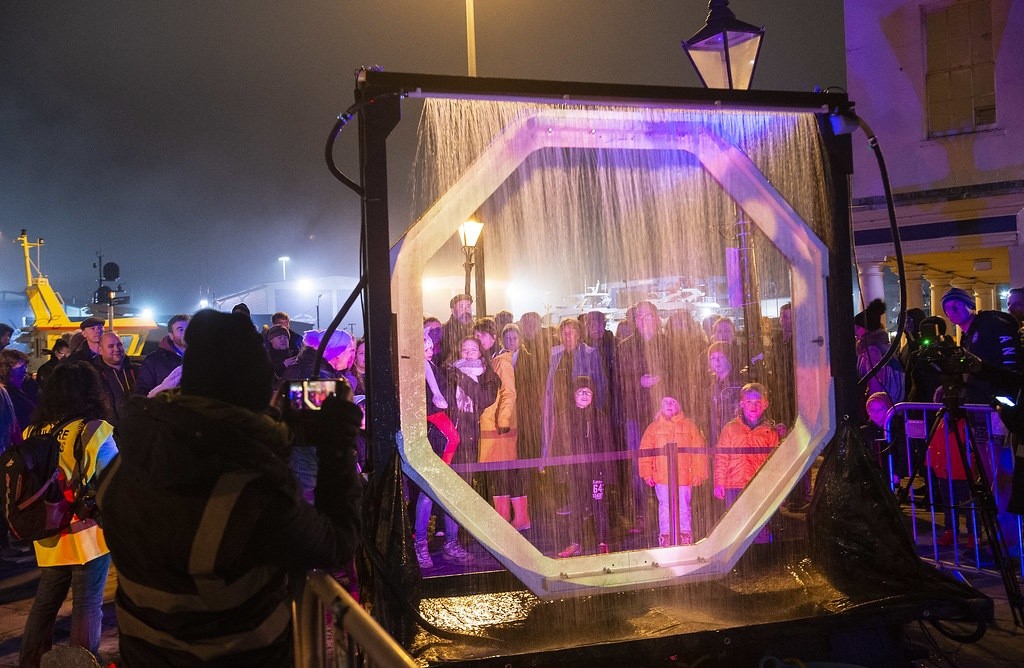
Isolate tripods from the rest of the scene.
[898,372,1024,632]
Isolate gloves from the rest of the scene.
[319,399,363,450]
[498,427,511,436]
[442,364,462,383]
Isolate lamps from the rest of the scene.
[973,259,992,270]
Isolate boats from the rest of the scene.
[0,227,156,377]
[536,280,720,336]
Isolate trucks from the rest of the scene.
[695,275,792,319]
[200,276,365,340]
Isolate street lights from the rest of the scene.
[459,212,484,296]
[279,255,292,281]
[348,323,356,333]
[317,294,323,330]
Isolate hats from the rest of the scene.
[423,329,434,351]
[302,328,352,363]
[707,341,734,365]
[941,287,975,319]
[866,391,892,412]
[267,324,290,341]
[180,309,274,415]
[853,298,885,331]
[80,316,105,330]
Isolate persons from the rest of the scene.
[415,294,811,571]
[857,287,1024,570]
[0,303,365,668]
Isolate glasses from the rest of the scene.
[575,389,592,396]
[57,350,66,356]
[635,314,658,321]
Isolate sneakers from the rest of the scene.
[443,539,477,565]
[414,538,433,567]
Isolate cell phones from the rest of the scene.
[995,396,1015,407]
[284,378,344,415]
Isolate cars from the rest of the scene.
[722,308,747,331]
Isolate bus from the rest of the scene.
[588,275,689,310]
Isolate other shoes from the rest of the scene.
[494,494,693,557]
[893,475,901,490]
[938,528,960,546]
[914,485,929,496]
[968,534,982,549]
[786,498,811,509]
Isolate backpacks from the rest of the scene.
[1,415,81,541]
